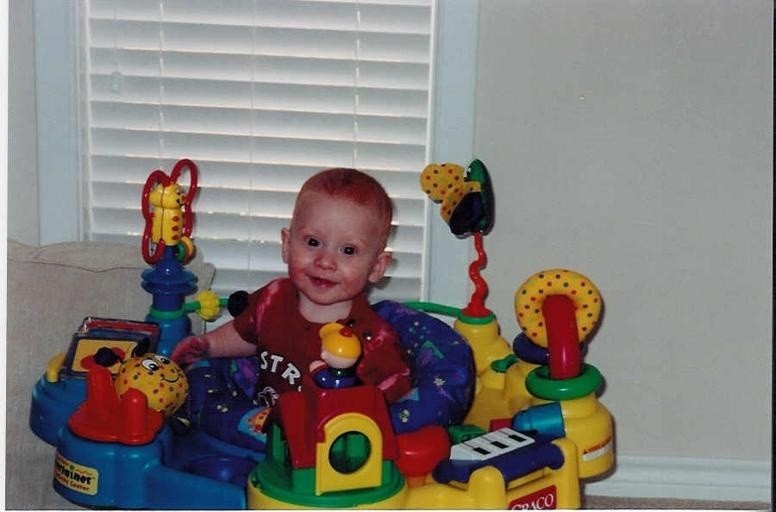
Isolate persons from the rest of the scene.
[169,166,416,410]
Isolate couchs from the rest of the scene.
[6,237,216,510]
